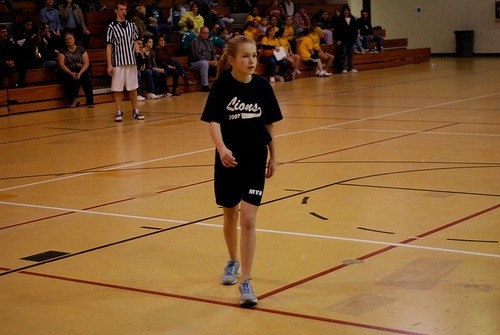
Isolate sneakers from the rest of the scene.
[239,277,258,306]
[132,108,146,119]
[115,110,123,121]
[220,260,240,284]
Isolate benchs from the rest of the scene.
[0,0,431,117]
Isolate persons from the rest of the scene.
[106,1,145,121]
[0,0,106,108]
[200,35,283,305]
[130,0,382,101]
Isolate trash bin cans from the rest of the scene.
[454,30,475,56]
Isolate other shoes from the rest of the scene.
[315,71,320,76]
[8,81,23,88]
[136,95,145,100]
[171,92,180,96]
[146,93,160,99]
[268,76,275,82]
[275,74,285,82]
[284,72,295,81]
[349,69,358,73]
[352,46,384,54]
[341,69,347,74]
[70,100,78,107]
[318,70,332,77]
[201,85,211,92]
[88,104,95,108]
[160,92,172,98]
[184,80,197,86]
